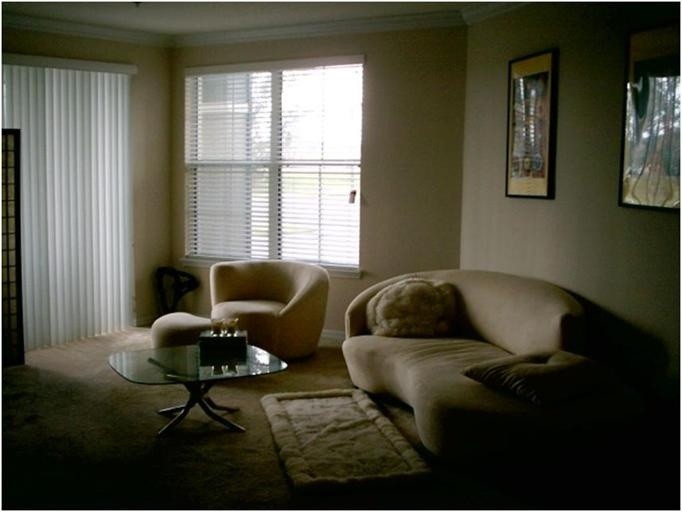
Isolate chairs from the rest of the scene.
[207,257,332,361]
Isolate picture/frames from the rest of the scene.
[503,23,679,212]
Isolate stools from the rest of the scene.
[149,311,211,377]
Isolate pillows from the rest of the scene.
[365,277,458,339]
[459,345,610,410]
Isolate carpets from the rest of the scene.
[259,386,434,489]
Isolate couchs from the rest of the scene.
[341,267,645,470]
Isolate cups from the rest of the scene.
[211,318,224,334]
[228,364,237,375]
[212,364,225,375]
[225,318,240,333]
